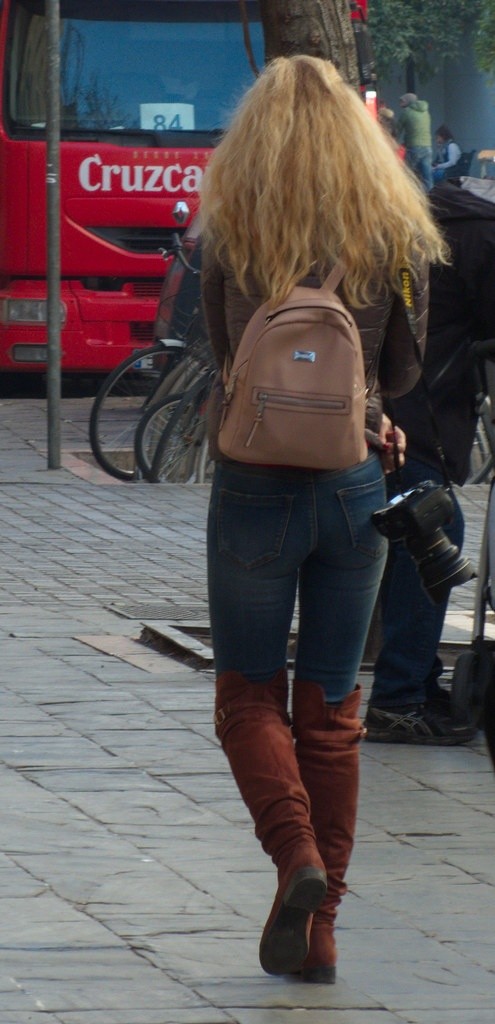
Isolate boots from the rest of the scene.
[215,666,327,976]
[289,681,366,984]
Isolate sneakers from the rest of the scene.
[363,686,479,745]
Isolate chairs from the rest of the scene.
[448,149,476,176]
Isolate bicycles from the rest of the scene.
[86,232,222,484]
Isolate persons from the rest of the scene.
[361,151,495,743]
[434,126,463,184]
[377,103,395,136]
[394,92,433,192]
[198,54,452,987]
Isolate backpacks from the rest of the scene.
[217,238,368,469]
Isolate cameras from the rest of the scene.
[369,482,479,607]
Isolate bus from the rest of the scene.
[1,0,382,396]
